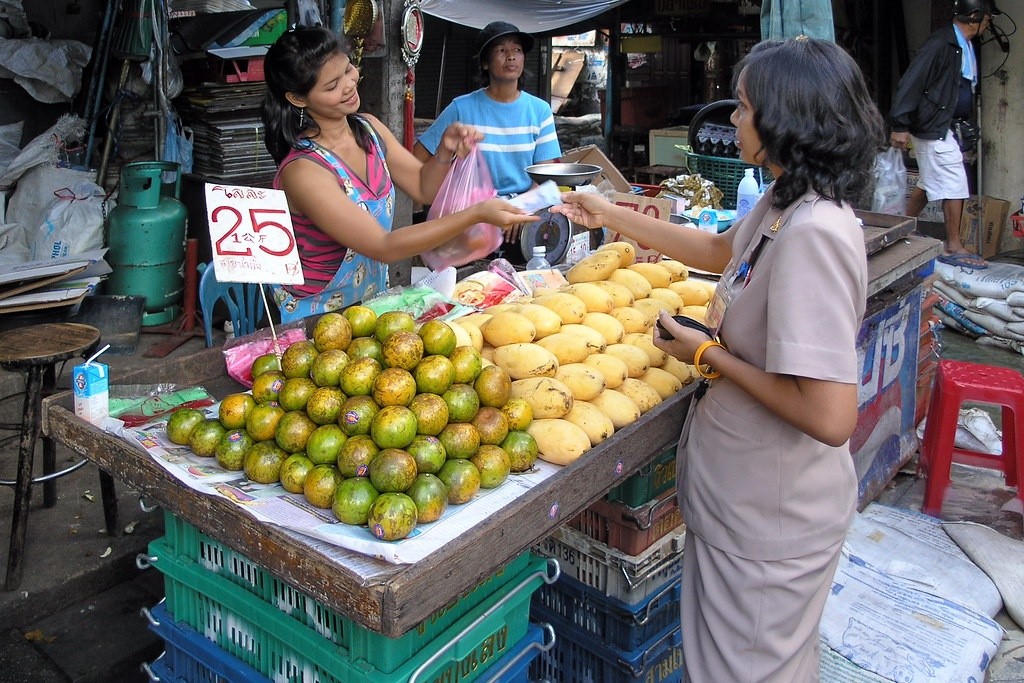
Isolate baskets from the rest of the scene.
[684,152,774,210]
[538,445,689,683]
[133,497,559,683]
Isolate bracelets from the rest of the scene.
[435,145,457,162]
[695,340,728,379]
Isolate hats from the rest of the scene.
[478,21,533,59]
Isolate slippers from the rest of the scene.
[938,253,989,269]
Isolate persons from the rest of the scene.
[262,26,542,327]
[547,39,887,683]
[413,22,562,262]
[891,0,1003,269]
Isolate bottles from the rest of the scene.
[526,246,551,270]
[737,168,759,221]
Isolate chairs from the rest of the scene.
[199,259,268,348]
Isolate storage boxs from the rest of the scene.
[561,144,635,193]
[40,209,942,682]
[906,168,945,223]
[959,195,1010,260]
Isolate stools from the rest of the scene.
[917,360,1024,517]
[610,129,648,182]
[634,165,687,185]
[607,0,892,169]
[0,323,122,590]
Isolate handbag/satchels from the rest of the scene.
[873,146,908,217]
[953,118,978,152]
[420,137,504,271]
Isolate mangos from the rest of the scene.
[449,241,718,468]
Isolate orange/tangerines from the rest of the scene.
[165,304,538,539]
[431,204,490,259]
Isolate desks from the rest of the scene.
[598,86,670,136]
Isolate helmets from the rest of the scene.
[954,0,1002,15]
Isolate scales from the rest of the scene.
[521,164,603,266]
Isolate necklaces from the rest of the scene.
[769,214,782,234]
